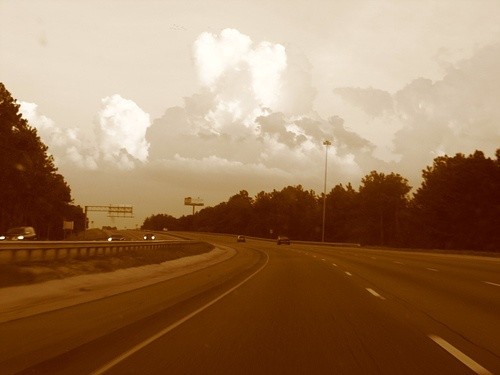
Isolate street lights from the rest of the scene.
[321,138,332,242]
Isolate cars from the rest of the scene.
[0,226,36,241]
[144,233,155,240]
[237,235,245,241]
[277,236,290,245]
[108,233,124,241]
